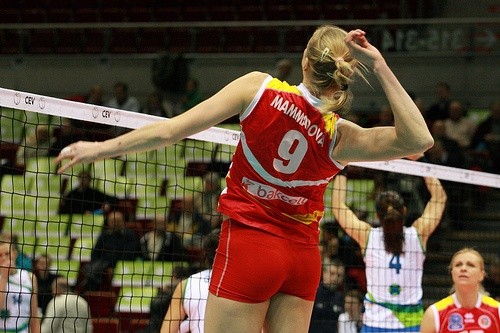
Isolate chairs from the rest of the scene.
[2,96,492,332]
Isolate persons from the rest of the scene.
[0,47,499,316]
[335,289,366,333]
[330,160,446,332]
[420,247,500,333]
[0,236,41,333]
[159,227,223,333]
[55,24,434,333]
[307,258,351,333]
[39,277,93,333]
